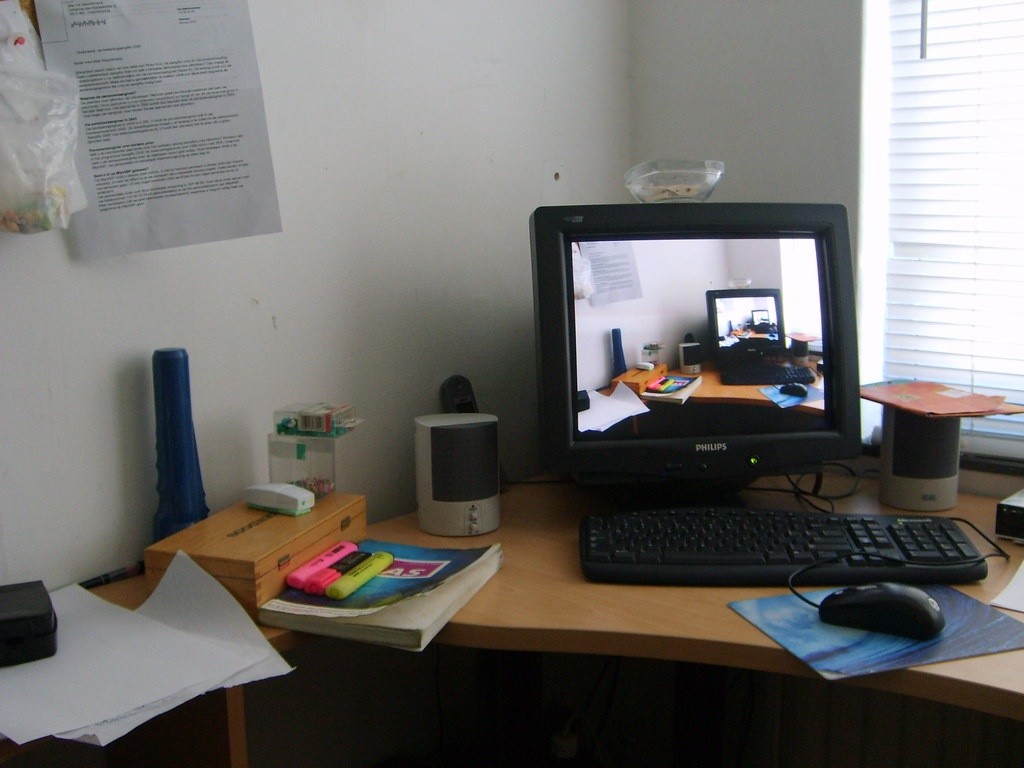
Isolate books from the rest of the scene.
[256,537,503,653]
[639,375,704,406]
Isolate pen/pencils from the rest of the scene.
[79,560,146,588]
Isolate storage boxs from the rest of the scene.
[612,363,667,396]
[144,492,367,623]
[730,329,743,337]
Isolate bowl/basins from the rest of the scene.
[622,157,724,203]
[729,279,752,289]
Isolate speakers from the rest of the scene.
[877,403,961,510]
[679,342,702,374]
[415,413,501,535]
[791,338,809,362]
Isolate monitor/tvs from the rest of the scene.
[706,289,786,359]
[529,203,861,509]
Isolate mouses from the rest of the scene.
[780,383,808,397]
[819,582,946,640]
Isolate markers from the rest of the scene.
[648,375,673,392]
[287,539,394,600]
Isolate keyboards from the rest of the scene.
[721,367,815,386]
[580,507,991,586]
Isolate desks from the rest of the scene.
[720,329,778,347]
[0,482,1024,768]
[578,360,825,434]
